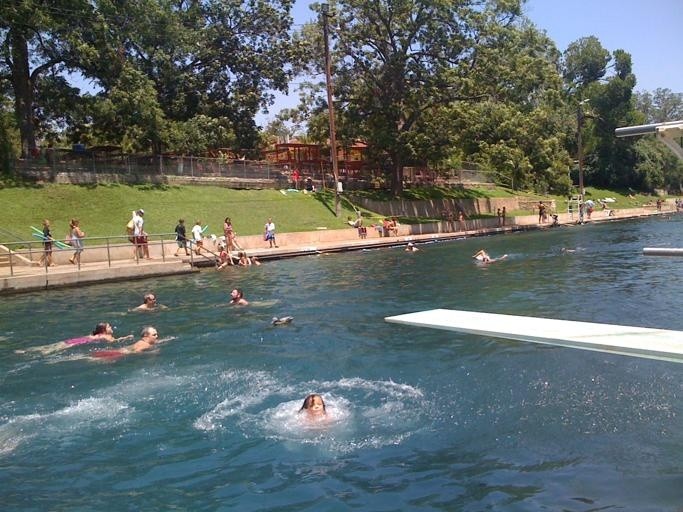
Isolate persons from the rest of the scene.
[348,216,363,227]
[675,198,682,208]
[457,211,467,232]
[446,208,456,232]
[69,219,84,266]
[502,207,506,226]
[405,243,420,252]
[293,167,301,190]
[497,209,502,226]
[326,174,333,188]
[264,218,279,247]
[174,217,261,269]
[568,196,615,226]
[37,220,54,266]
[657,199,667,211]
[471,247,508,264]
[355,207,361,218]
[125,208,152,261]
[538,200,559,226]
[297,395,332,426]
[562,246,587,253]
[306,177,315,192]
[19,289,305,374]
[370,216,399,237]
[337,179,343,193]
